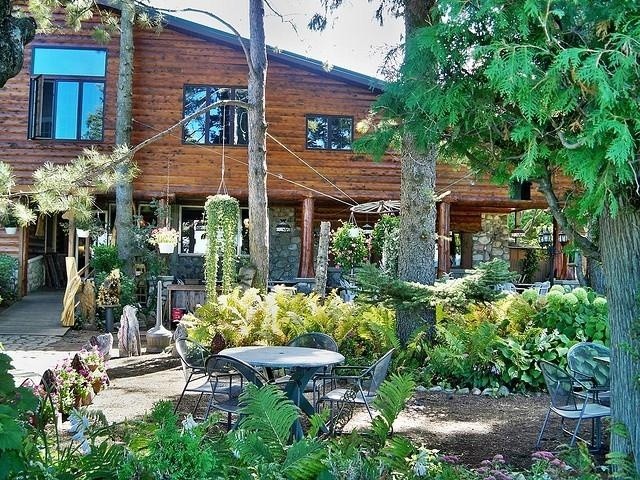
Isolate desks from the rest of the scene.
[593,357,611,365]
[222,346,340,402]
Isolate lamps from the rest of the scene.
[538,225,554,256]
[553,230,569,254]
[276,219,290,233]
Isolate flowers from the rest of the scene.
[148,224,180,244]
[35,340,109,413]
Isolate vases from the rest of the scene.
[157,244,176,254]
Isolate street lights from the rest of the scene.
[539,226,570,289]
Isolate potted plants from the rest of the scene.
[1,199,34,235]
[68,189,98,238]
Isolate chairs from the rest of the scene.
[204,353,302,432]
[562,344,614,445]
[311,347,395,447]
[274,335,337,413]
[175,337,242,422]
[534,354,609,455]
[494,280,550,295]
